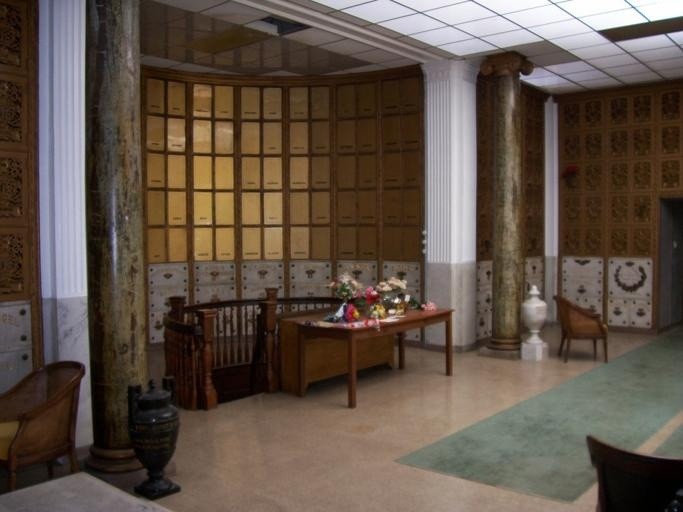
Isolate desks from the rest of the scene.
[276,293,453,410]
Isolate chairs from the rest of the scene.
[584,433,682,510]
[0,354,92,489]
[551,293,610,364]
